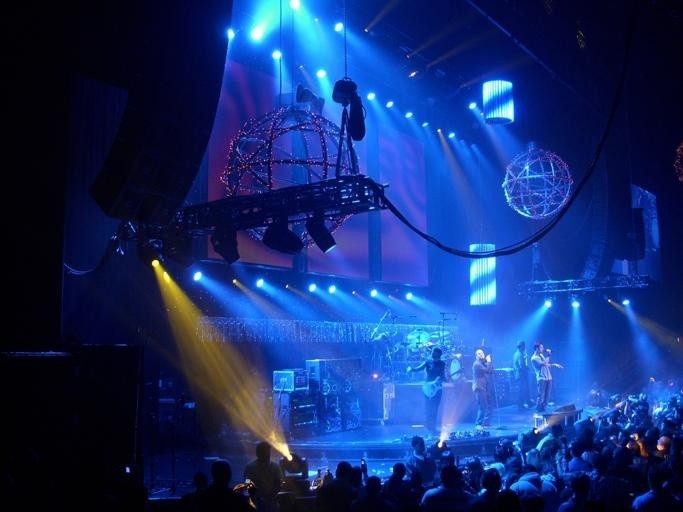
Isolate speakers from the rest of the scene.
[577,104,617,297]
[89,0,234,227]
[0,344,143,512]
[545,404,579,428]
[0,0,72,354]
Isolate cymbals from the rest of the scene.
[430,331,449,338]
[407,331,429,342]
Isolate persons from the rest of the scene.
[472,349,492,431]
[184,378,683,512]
[530,344,562,412]
[406,347,450,431]
[512,340,537,410]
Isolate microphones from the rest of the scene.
[544,349,552,353]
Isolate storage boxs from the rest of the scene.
[273,370,310,391]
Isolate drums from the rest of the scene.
[446,359,462,383]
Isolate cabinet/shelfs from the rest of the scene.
[272,392,320,440]
[492,368,520,407]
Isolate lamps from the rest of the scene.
[211,223,240,264]
[427,441,451,462]
[136,226,168,272]
[263,216,305,255]
[280,453,311,487]
[482,80,515,126]
[220,0,359,248]
[526,428,540,441]
[169,229,208,267]
[469,244,496,306]
[502,142,573,219]
[305,211,336,253]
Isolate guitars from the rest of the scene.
[422,368,464,400]
